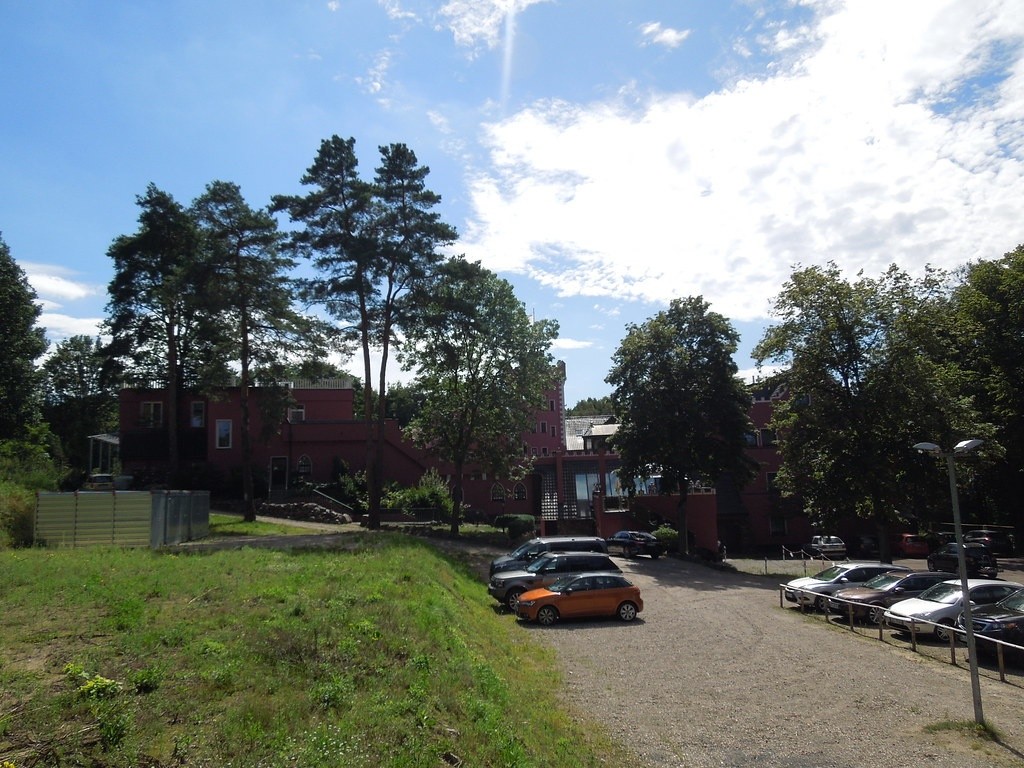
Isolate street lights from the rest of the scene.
[914,439,985,725]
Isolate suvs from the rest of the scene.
[923,531,958,556]
[490,535,608,576]
[928,543,998,578]
[961,529,1011,556]
[488,550,623,613]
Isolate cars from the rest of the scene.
[802,535,847,559]
[883,578,1023,640]
[605,530,665,560]
[785,563,913,611]
[515,572,644,627]
[848,535,881,559]
[827,570,962,625]
[953,587,1024,662]
[888,532,930,558]
